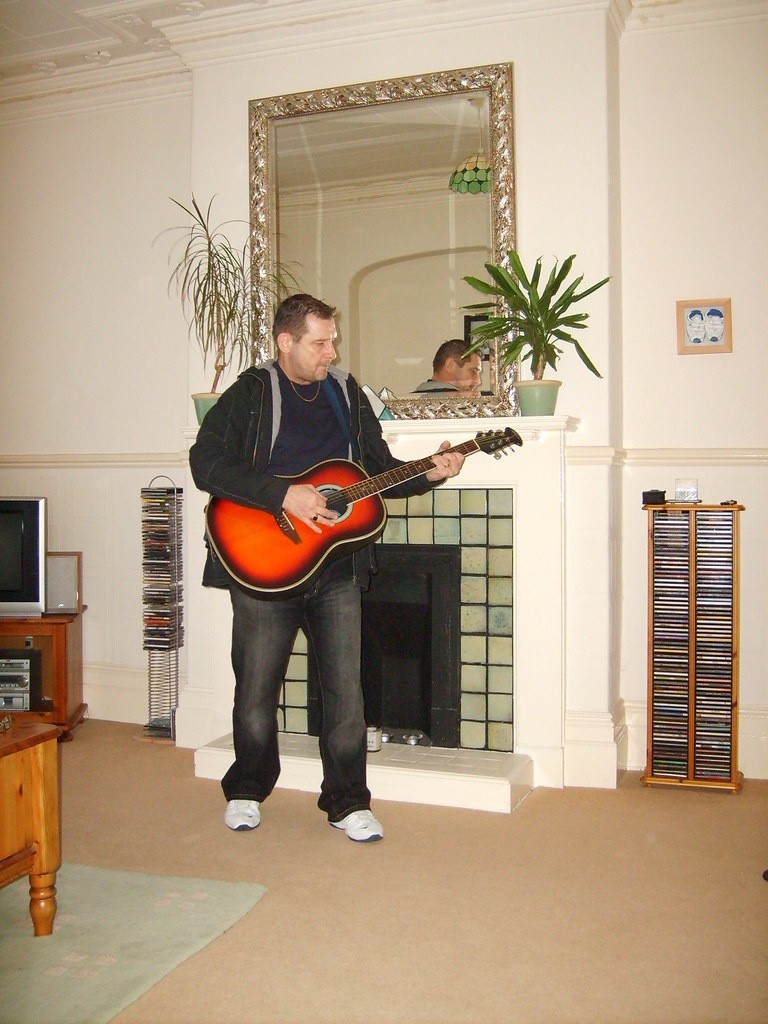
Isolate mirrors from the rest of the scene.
[241,60,521,419]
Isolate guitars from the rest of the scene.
[204,425,524,600]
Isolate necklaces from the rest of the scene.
[276,360,321,402]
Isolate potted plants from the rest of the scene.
[461,249,614,416]
[151,193,307,424]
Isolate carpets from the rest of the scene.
[0,860,269,1024]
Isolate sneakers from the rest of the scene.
[330,809,383,841]
[225,799,261,830]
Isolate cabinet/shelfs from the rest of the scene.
[0,723,62,938]
[140,474,185,742]
[0,606,89,742]
[640,505,747,795]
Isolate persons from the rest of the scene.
[189,294,465,841]
[411,339,482,393]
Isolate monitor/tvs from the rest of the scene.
[0,495,47,619]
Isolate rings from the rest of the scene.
[313,513,318,521]
[444,464,449,467]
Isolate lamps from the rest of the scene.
[447,98,491,193]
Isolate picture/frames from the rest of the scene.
[463,314,491,360]
[676,297,733,355]
